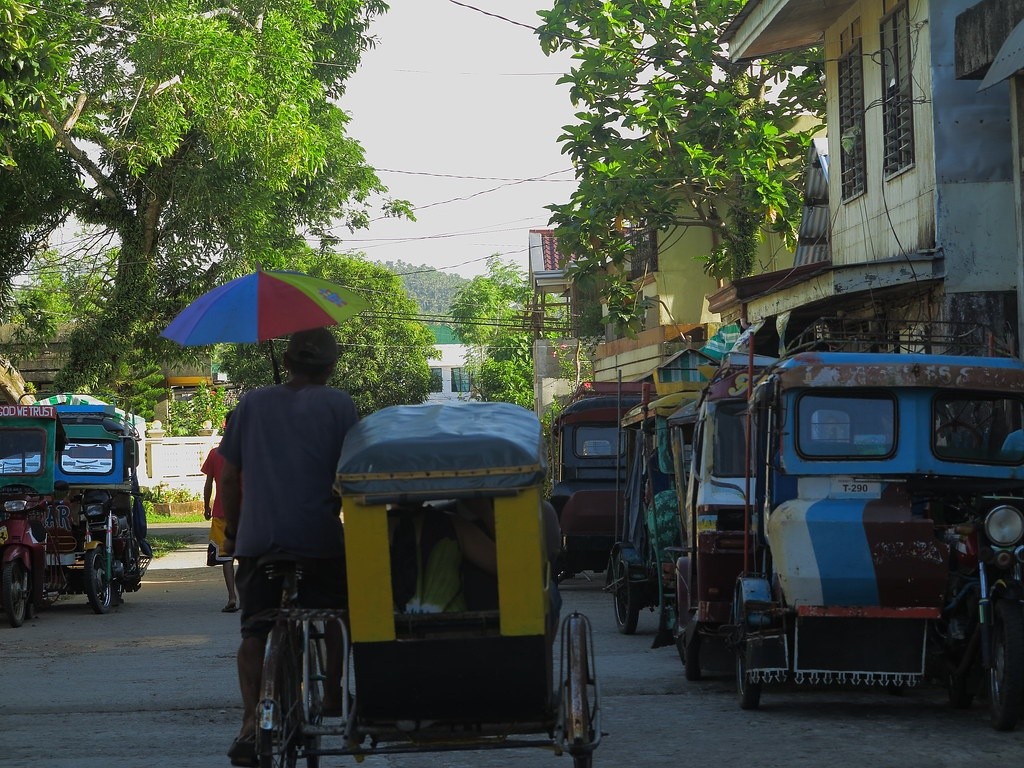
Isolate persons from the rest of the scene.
[201,411,242,612]
[217,327,360,757]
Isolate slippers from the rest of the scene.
[221,603,239,612]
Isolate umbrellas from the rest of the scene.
[160,262,373,385]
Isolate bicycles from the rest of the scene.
[231,547,346,768]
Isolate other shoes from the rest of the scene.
[227,714,260,757]
[321,689,357,717]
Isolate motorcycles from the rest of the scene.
[911,490,1024,731]
[0,493,70,628]
[54,480,154,614]
